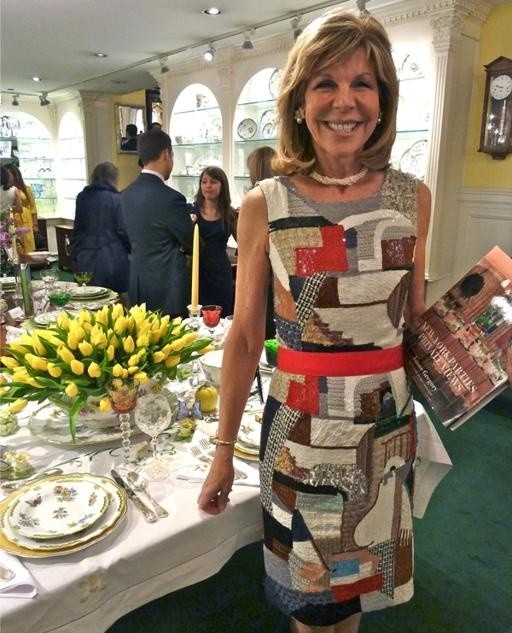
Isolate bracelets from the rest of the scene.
[212,436,239,447]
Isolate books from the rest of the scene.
[402,244,512,432]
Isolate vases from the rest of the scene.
[61,388,155,429]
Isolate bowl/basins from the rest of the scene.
[26,251,51,261]
[264,338,279,367]
[199,350,225,387]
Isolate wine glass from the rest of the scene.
[201,304,223,338]
[47,288,74,316]
[135,391,172,479]
[73,270,95,289]
[40,268,61,287]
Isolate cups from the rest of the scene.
[225,315,236,337]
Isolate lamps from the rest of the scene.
[12,94,20,105]
[160,0,370,74]
[38,92,50,105]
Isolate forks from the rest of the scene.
[200,437,248,481]
[189,445,238,479]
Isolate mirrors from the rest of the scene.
[113,101,146,154]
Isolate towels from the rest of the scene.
[0,549,39,598]
[176,429,260,487]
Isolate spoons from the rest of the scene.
[126,470,168,518]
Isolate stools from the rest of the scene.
[55,224,74,271]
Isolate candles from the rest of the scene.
[191,224,199,305]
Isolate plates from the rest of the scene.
[399,53,421,80]
[232,443,259,454]
[233,70,287,140]
[1,472,128,557]
[257,360,272,374]
[64,284,120,311]
[0,277,19,294]
[393,139,430,186]
[26,392,181,447]
[229,449,259,462]
[238,409,286,446]
[33,309,84,328]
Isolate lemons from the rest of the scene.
[194,385,219,412]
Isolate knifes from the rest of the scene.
[110,468,155,522]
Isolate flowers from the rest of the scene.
[0,302,211,408]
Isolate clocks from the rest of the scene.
[477,55,512,159]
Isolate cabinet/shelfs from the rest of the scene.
[389,74,436,183]
[60,137,86,200]
[170,104,222,177]
[1,134,56,199]
[235,99,285,182]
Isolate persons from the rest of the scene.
[3,163,38,252]
[147,123,161,131]
[120,128,194,319]
[121,124,137,150]
[191,166,238,319]
[0,166,23,257]
[246,147,281,186]
[197,7,433,633]
[69,162,132,305]
